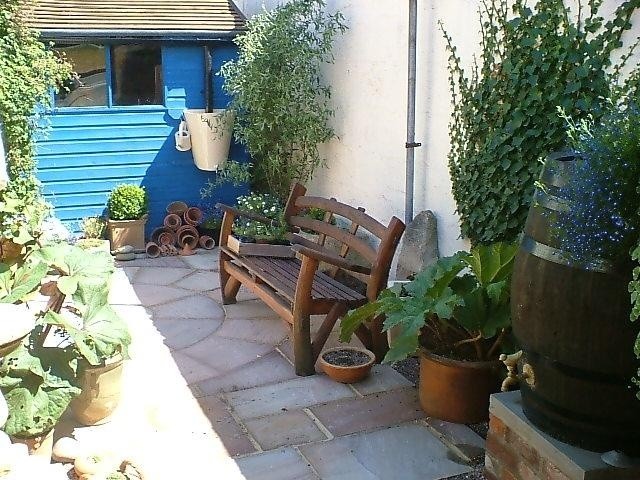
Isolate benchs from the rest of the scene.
[214,183,407,377]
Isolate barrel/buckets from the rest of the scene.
[506,153,639,453]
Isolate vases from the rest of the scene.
[146,199,215,258]
[239,235,277,245]
[320,346,376,384]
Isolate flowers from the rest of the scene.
[230,189,337,244]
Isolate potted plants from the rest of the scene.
[43,276,134,428]
[335,239,521,425]
[0,186,54,261]
[68,213,109,256]
[104,181,151,252]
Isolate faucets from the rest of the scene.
[496,350,535,394]
